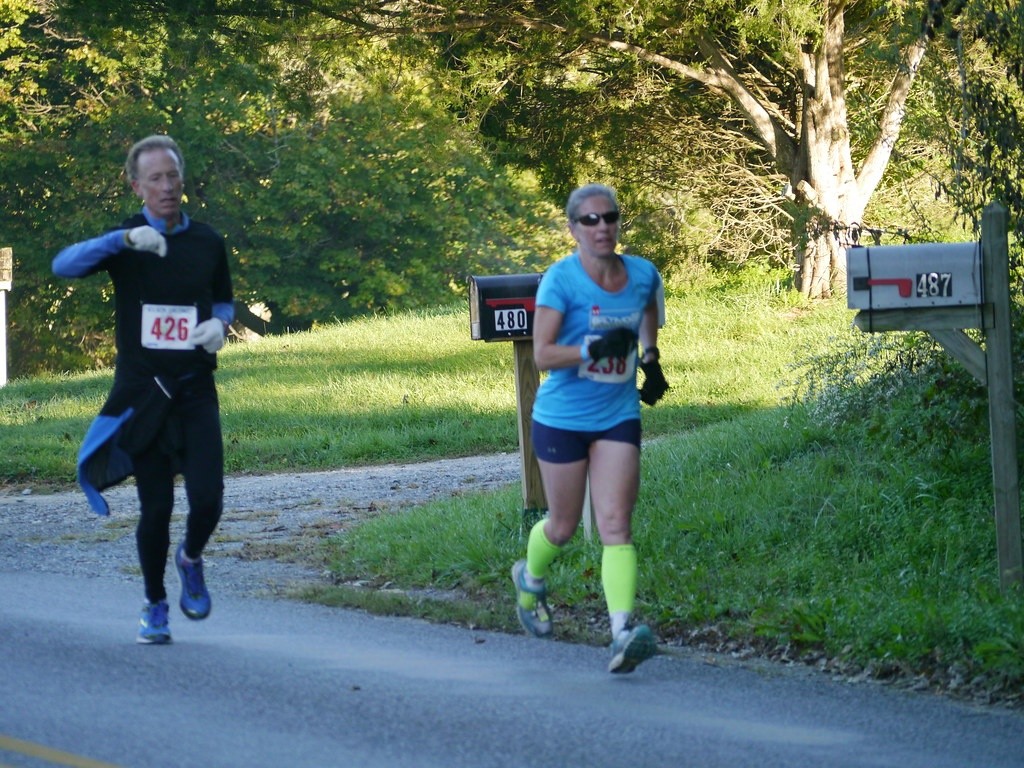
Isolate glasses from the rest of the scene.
[574,211,620,226]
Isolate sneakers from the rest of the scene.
[607,624,655,674]
[511,559,552,637]
[175,543,211,620]
[137,598,170,644]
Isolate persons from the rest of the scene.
[50,133,239,647]
[509,182,670,676]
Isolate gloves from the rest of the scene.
[640,361,670,406]
[589,327,638,362]
[191,317,224,354]
[129,225,168,258]
